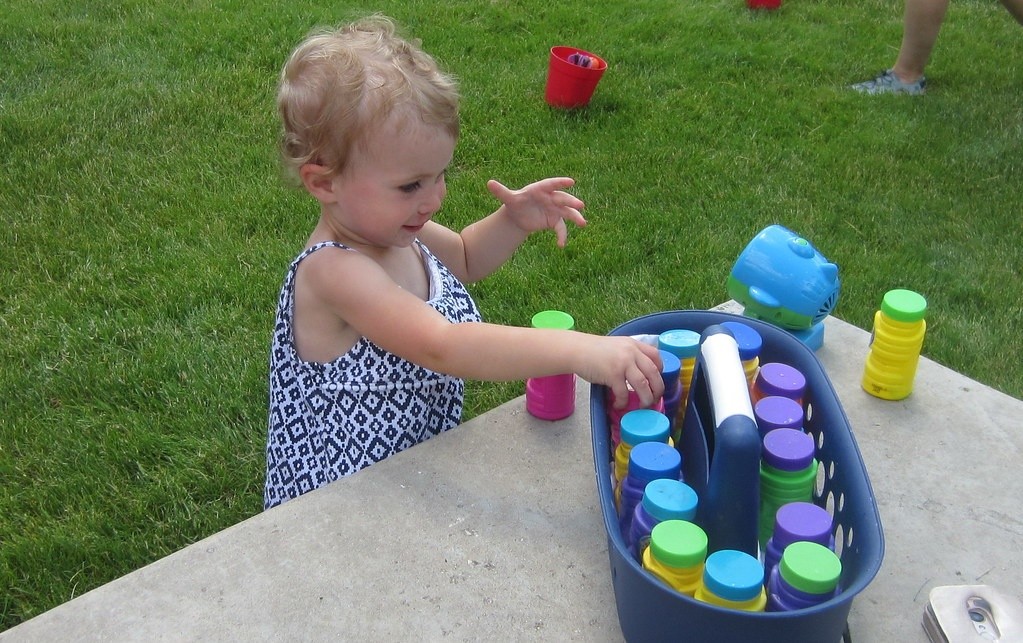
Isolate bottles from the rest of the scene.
[861,288,926,401]
[608,322,844,611]
[525,310,577,421]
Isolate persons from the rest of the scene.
[262,11,664,517]
[853,0,1022,95]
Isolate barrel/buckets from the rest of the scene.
[545,46,607,108]
[744,0,782,9]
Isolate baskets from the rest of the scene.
[590,310,886,643]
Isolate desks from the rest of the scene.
[0,292,1023,643]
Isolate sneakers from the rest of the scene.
[851,69,928,96]
